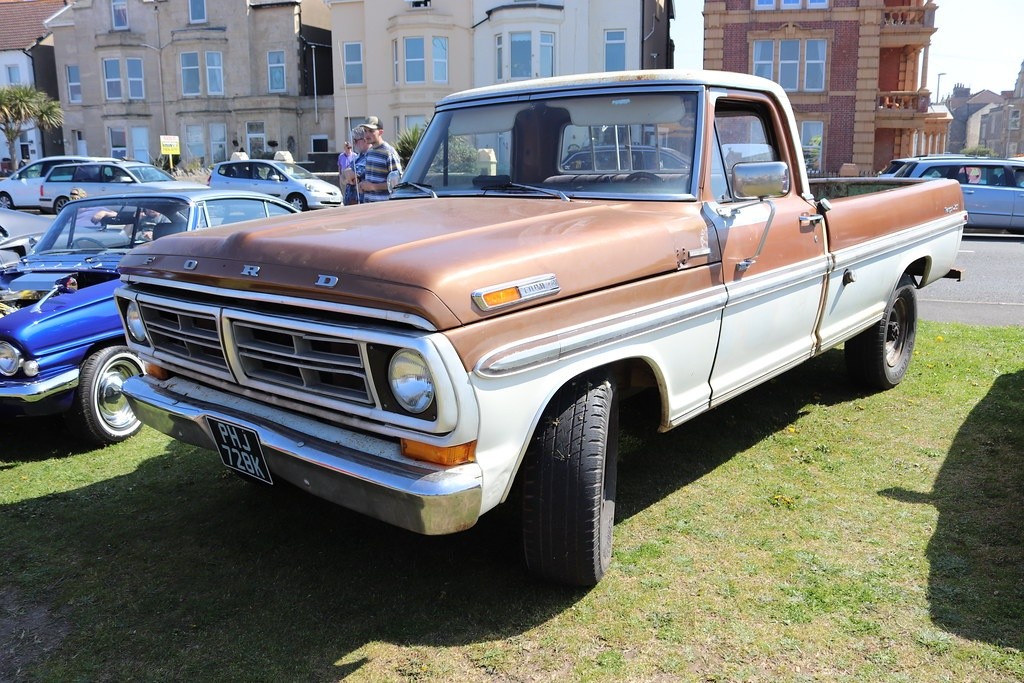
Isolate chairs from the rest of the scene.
[542,171,697,198]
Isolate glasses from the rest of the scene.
[347,146,352,150]
[353,138,364,144]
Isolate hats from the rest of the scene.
[360,116,383,129]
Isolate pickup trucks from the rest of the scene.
[113,68,969,593]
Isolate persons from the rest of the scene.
[109,170,122,182]
[357,116,403,203]
[343,124,373,205]
[1014,169,1024,187]
[337,141,357,194]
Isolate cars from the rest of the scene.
[0,155,121,214]
[39,159,211,215]
[208,157,345,211]
[906,158,1024,239]
[0,192,298,444]
[877,155,967,181]
